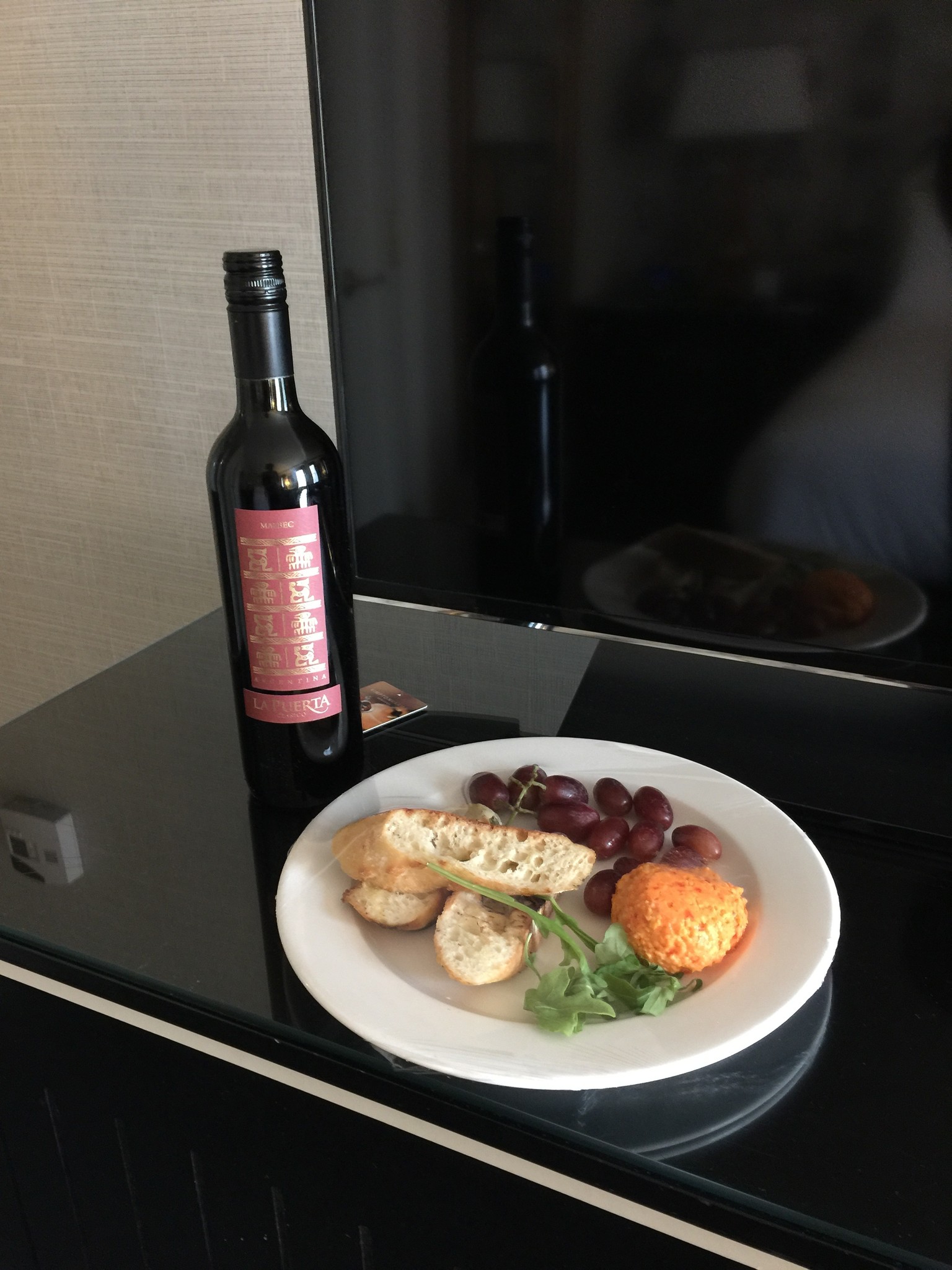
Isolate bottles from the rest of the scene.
[203,244,363,796]
[489,215,557,544]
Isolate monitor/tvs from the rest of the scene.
[303,0,952,850]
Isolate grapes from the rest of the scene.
[466,767,721,916]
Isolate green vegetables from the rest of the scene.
[420,860,703,1034]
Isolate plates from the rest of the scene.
[274,735,841,1092]
[580,526,929,654]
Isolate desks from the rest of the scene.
[0,593,952,1270]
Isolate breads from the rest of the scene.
[335,808,594,986]
